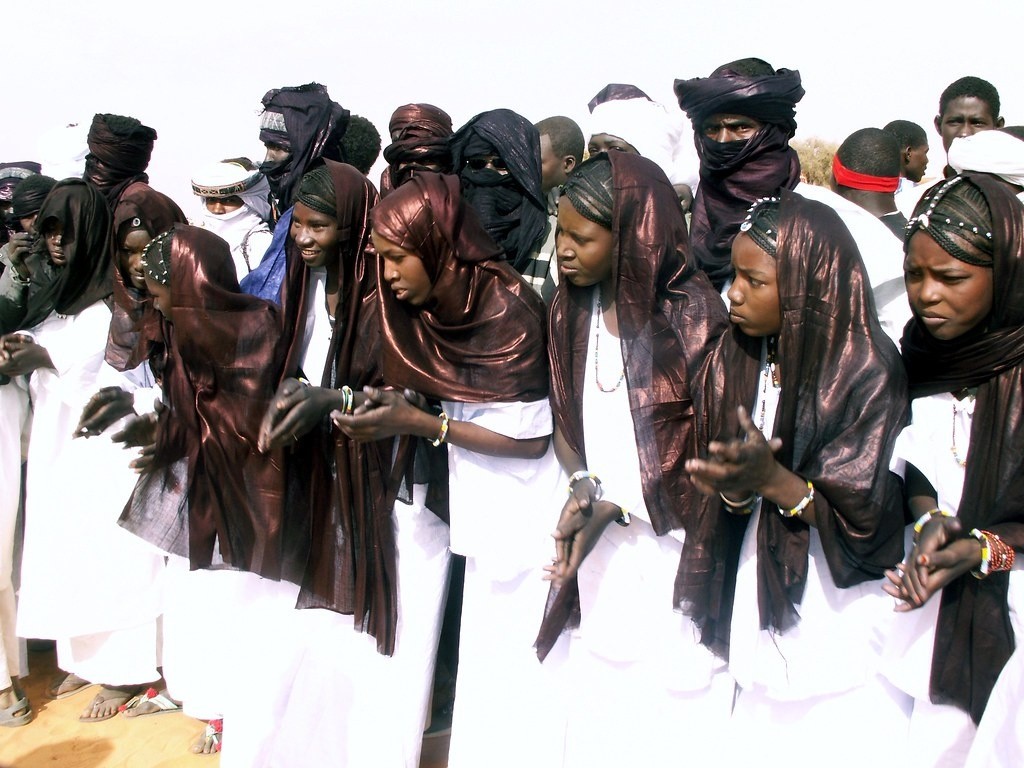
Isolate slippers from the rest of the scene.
[118,687,183,719]
[44,672,94,699]
[192,719,223,755]
[79,684,149,722]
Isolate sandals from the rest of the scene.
[0,696,34,727]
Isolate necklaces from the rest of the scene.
[771,349,781,393]
[951,405,966,467]
[595,294,625,391]
[771,344,784,431]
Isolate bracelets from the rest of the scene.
[568,471,603,501]
[615,507,631,527]
[432,412,448,446]
[912,509,949,546]
[342,386,353,415]
[339,389,345,413]
[969,528,991,579]
[980,530,1014,570]
[725,496,758,513]
[777,481,814,516]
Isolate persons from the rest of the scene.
[0,56,1024,768]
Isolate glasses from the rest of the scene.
[466,157,509,169]
[401,150,452,165]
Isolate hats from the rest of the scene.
[389,103,453,141]
[673,58,805,130]
[383,119,454,166]
[83,113,160,186]
[256,81,350,152]
[0,161,40,190]
